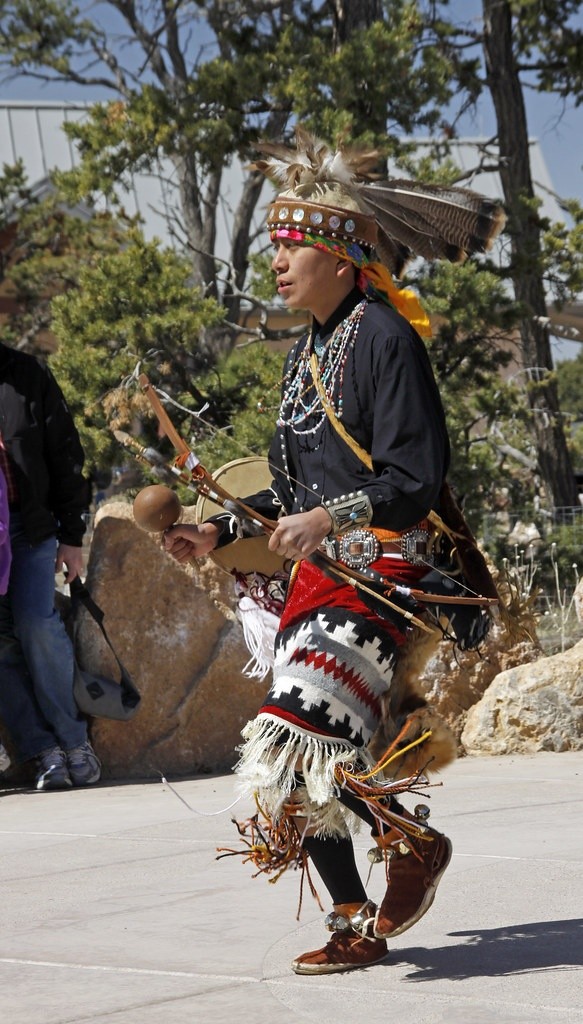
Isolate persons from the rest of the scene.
[161,179,453,977]
[0,340,102,791]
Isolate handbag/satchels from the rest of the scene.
[62,571,142,722]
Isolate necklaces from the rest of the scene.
[257,298,368,436]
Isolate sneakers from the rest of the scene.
[67,740,101,786]
[36,747,73,790]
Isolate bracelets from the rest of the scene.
[321,490,373,539]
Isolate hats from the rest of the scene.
[249,130,508,277]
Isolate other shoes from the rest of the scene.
[291,902,390,975]
[0,761,39,789]
[370,805,453,940]
